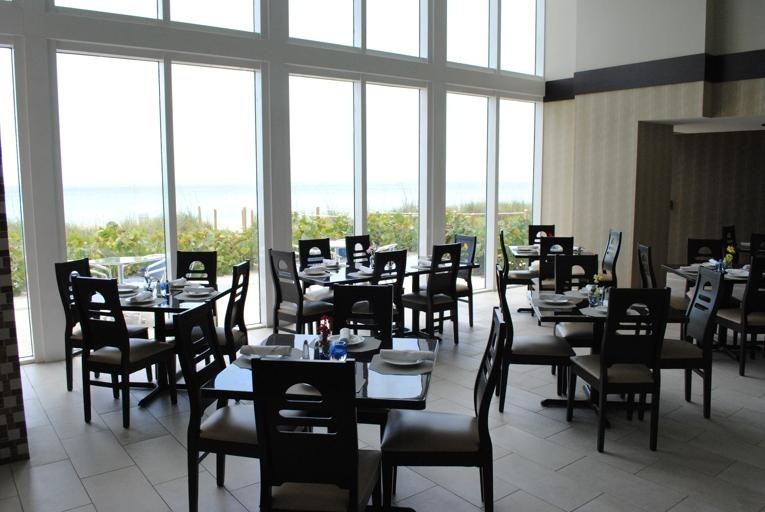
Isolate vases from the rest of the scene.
[314,332,332,360]
[588,288,600,307]
[143,283,155,293]
[579,228,622,288]
[719,262,727,272]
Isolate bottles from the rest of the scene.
[165,280,169,294]
[157,280,160,294]
[302,340,309,359]
[314,341,319,360]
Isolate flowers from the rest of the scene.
[124,265,159,288]
[319,316,333,357]
[592,274,604,300]
[723,246,735,268]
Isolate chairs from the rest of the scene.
[169,260,250,401]
[299,238,335,334]
[345,235,370,266]
[529,237,574,294]
[715,256,765,376]
[374,250,407,271]
[252,358,383,512]
[496,266,576,413]
[379,306,506,512]
[723,225,740,266]
[421,234,477,328]
[73,274,177,429]
[268,248,334,334]
[566,288,670,453]
[687,238,724,262]
[637,267,723,422]
[56,258,153,398]
[144,253,166,280]
[499,230,538,295]
[88,262,110,278]
[528,225,555,267]
[332,283,393,340]
[164,251,217,365]
[401,243,462,344]
[370,243,397,265]
[174,303,308,512]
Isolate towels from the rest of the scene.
[117,283,139,289]
[238,344,293,356]
[184,285,215,293]
[168,276,186,286]
[304,266,326,273]
[378,348,435,363]
[322,258,339,267]
[539,293,569,301]
[338,327,351,344]
[130,290,152,302]
[709,257,719,266]
[358,265,374,274]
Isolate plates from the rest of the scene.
[246,354,283,360]
[726,269,749,276]
[539,294,568,303]
[596,305,608,313]
[186,292,209,296]
[125,297,153,303]
[327,335,365,345]
[384,359,423,366]
